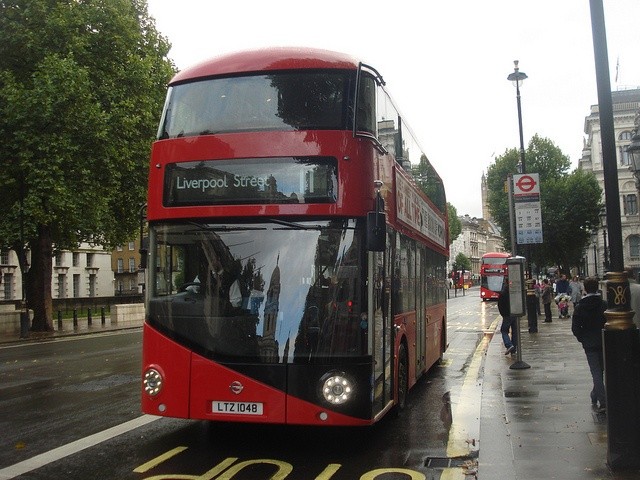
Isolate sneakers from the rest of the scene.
[505,346,514,356]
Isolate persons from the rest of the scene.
[555,275,586,319]
[571,278,607,411]
[178,248,243,308]
[624,265,640,329]
[542,278,553,323]
[497,276,519,357]
[449,282,453,289]
[532,280,542,316]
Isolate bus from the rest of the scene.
[141,46,449,429]
[480,252,511,302]
[448,270,472,288]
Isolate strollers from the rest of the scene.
[558,297,571,319]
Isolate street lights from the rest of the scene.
[599,205,610,276]
[590,230,600,292]
[507,60,538,334]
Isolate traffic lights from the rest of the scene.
[462,264,465,274]
[452,262,457,271]
